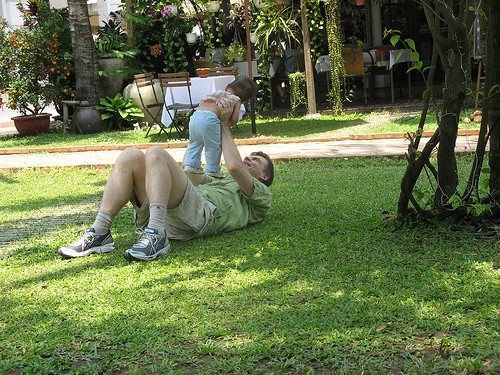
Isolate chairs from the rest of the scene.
[134,72,175,139]
[233,44,413,109]
[158,72,200,138]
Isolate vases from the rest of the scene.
[73,108,101,135]
[196,70,210,78]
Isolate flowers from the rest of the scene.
[196,68,210,70]
[146,6,172,19]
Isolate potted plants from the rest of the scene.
[0,0,75,134]
[95,19,138,97]
[119,0,364,117]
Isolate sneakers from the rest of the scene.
[58,227,116,258]
[124,227,171,261]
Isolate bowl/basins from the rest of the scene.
[196,68,210,78]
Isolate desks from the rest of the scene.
[315,49,414,104]
[161,75,246,128]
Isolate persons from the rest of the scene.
[58,98,274,262]
[184,75,257,178]
